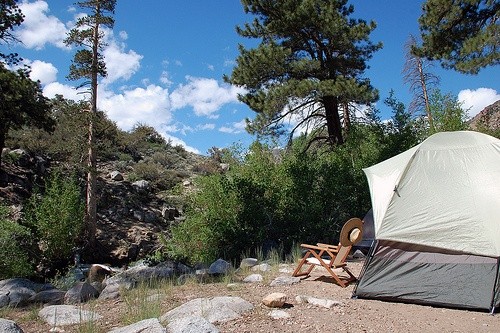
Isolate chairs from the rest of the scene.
[291,218,365,289]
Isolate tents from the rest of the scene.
[351,130,500,313]
[350,206,378,261]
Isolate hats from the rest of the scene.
[340,218,365,249]
